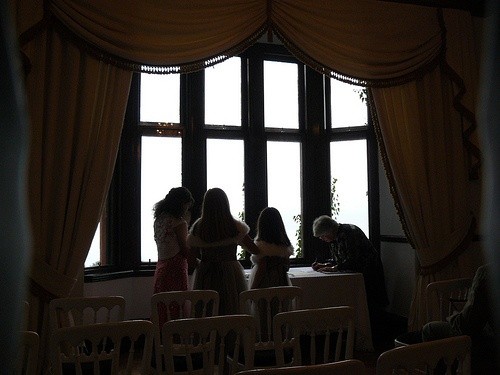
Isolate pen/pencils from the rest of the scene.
[314,256,318,262]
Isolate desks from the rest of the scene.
[239,265,373,358]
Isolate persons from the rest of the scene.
[250,208,290,344]
[149,187,199,370]
[182,189,260,364]
[312,215,390,350]
[421,263,500,375]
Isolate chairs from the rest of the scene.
[13,273,491,375]
